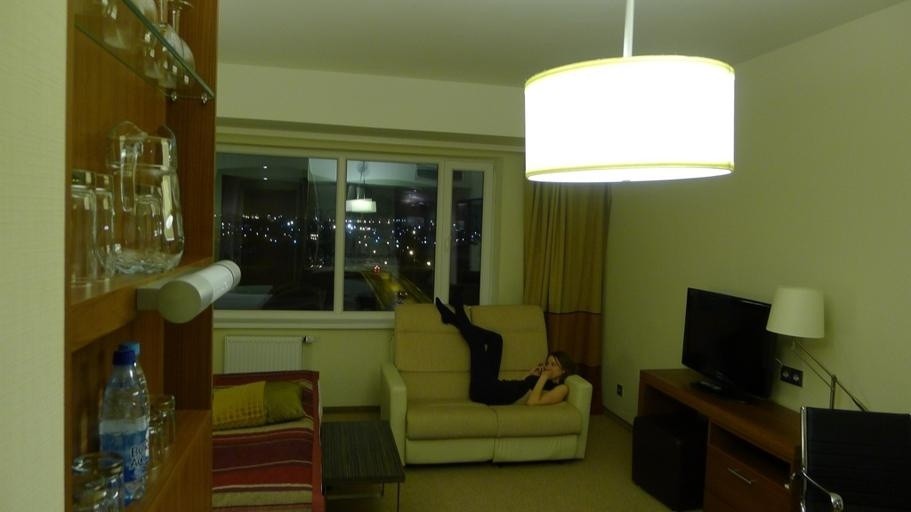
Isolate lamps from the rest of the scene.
[346,163,377,219]
[766,285,868,410]
[523,1,736,182]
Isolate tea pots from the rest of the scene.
[93,112,185,276]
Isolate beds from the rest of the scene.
[212,371,324,511]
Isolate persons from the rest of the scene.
[435,297,570,407]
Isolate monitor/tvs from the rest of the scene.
[681,287,779,403]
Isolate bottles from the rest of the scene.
[121,340,151,461]
[98,350,147,502]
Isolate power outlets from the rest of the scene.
[615,385,625,397]
[778,365,803,387]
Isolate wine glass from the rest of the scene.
[103,1,156,50]
[172,2,197,87]
[143,0,183,82]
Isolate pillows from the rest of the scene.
[266,381,306,425]
[211,381,265,429]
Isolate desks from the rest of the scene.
[320,419,406,512]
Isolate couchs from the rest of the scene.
[381,303,593,467]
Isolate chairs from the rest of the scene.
[801,404,910,511]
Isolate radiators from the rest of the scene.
[223,335,313,375]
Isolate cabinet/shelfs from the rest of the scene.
[2,0,219,512]
[631,369,800,512]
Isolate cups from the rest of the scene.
[91,172,119,280]
[71,452,123,510]
[148,411,164,469]
[71,170,97,286]
[148,391,177,447]
